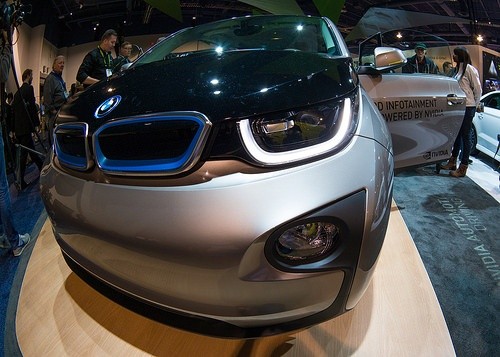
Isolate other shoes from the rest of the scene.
[20,181,31,187]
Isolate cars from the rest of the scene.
[470,90,500,163]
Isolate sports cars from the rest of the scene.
[39,14,468,330]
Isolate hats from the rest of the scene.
[415,42,426,49]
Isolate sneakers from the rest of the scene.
[0,234,12,247]
[14,233,29,257]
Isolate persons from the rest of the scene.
[66,84,77,98]
[440,45,484,178]
[437,61,452,75]
[114,42,133,73]
[75,29,118,89]
[0,90,13,177]
[42,55,68,149]
[402,41,435,75]
[7,69,45,192]
[0,131,31,256]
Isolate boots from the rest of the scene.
[450,164,468,177]
[441,157,456,170]
